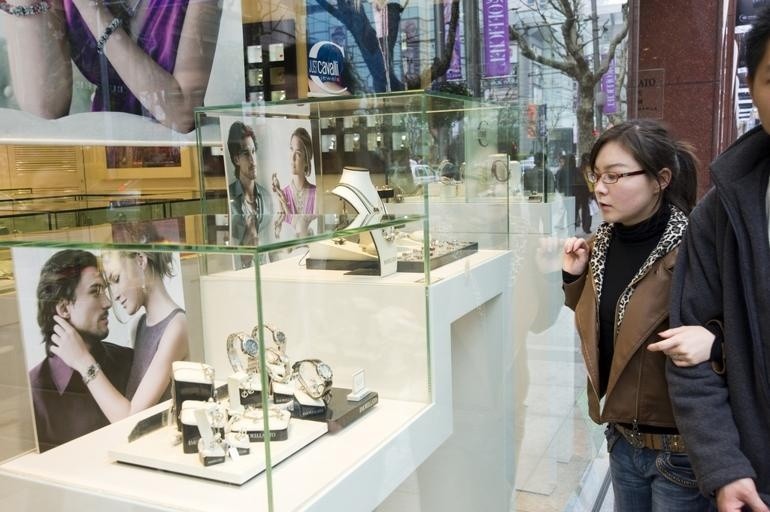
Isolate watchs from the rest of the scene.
[292,359,336,400]
[222,329,260,385]
[251,320,289,364]
[260,347,292,385]
[82,361,101,384]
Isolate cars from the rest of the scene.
[519,159,536,172]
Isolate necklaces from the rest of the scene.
[118,0,144,18]
[173,359,216,382]
[333,181,396,242]
[291,179,308,213]
[293,215,310,231]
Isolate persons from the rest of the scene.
[659,9,770,511]
[230,215,274,269]
[27,248,136,453]
[0,0,226,135]
[557,115,728,511]
[45,219,189,427]
[524,146,597,236]
[225,120,275,215]
[271,126,318,214]
[275,215,319,242]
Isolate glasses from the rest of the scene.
[585,169,648,186]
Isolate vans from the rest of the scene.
[384,164,440,195]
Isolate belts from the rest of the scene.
[614,422,685,458]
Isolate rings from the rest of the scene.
[204,405,247,453]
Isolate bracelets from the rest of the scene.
[240,404,286,424]
[0,0,54,17]
[94,17,123,55]
